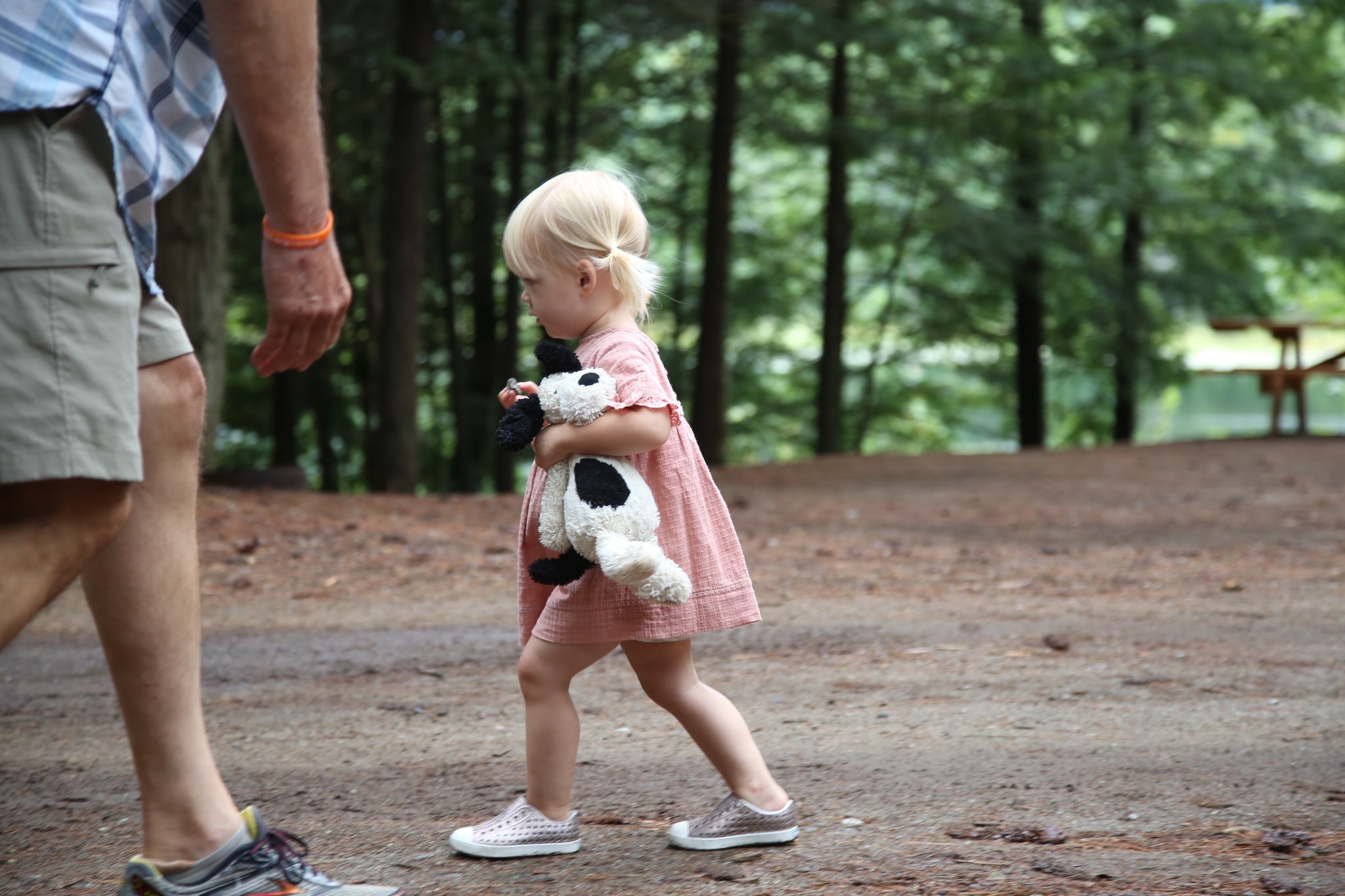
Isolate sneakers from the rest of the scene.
[447,796,583,859]
[117,804,406,896]
[666,793,800,850]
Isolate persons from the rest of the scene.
[0,1,400,896]
[445,167,801,856]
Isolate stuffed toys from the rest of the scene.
[491,343,692,606]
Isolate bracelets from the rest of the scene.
[262,209,334,247]
